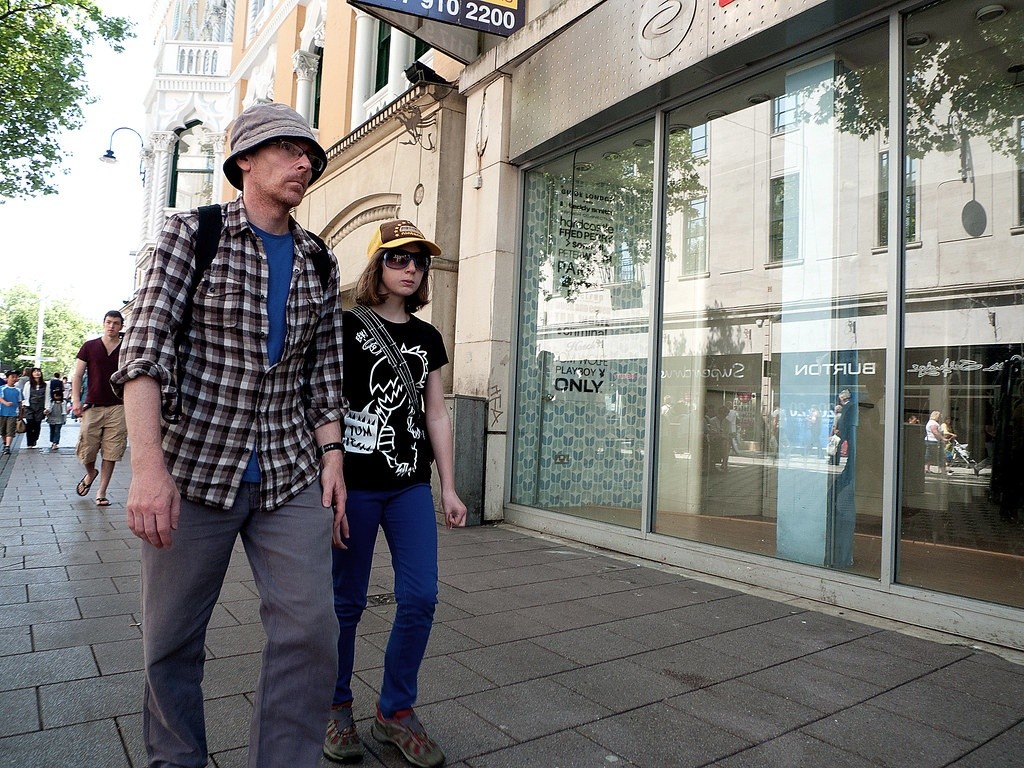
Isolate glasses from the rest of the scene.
[264,140,323,171]
[379,250,431,272]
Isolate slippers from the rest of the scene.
[76,469,99,496]
[96,497,112,505]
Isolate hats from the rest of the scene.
[222,104,328,191]
[368,219,442,261]
[6,369,21,378]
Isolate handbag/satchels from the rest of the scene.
[16,418,25,433]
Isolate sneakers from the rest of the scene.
[323,701,364,760]
[369,699,446,768]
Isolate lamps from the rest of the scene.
[404,60,450,84]
[98,127,146,188]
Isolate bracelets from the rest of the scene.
[318,443,346,459]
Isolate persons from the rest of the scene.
[47,373,72,403]
[0,370,23,455]
[660,389,993,475]
[110,105,347,767]
[22,368,49,449]
[320,218,469,768]
[46,389,67,450]
[19,367,30,390]
[827,377,920,520]
[71,310,126,506]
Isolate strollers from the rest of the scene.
[947,435,978,468]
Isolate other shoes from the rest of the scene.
[52,444,59,450]
[4,446,10,455]
